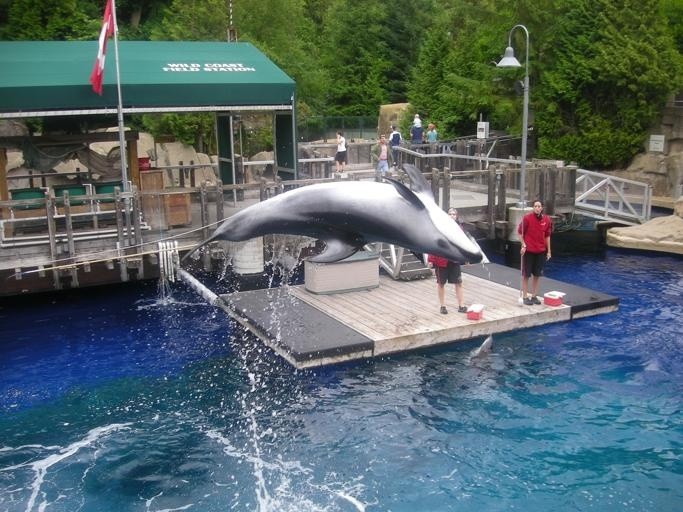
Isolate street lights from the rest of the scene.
[495,24,530,209]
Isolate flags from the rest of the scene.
[89,0,114,96]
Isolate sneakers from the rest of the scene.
[440,306,448,314]
[523,297,541,305]
[458,306,468,312]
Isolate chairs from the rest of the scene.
[8,181,125,237]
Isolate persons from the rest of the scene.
[426,123,437,144]
[371,135,392,184]
[388,125,402,165]
[335,131,347,172]
[410,113,425,144]
[517,200,552,305]
[427,208,467,314]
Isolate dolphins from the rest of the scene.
[177,160,484,267]
